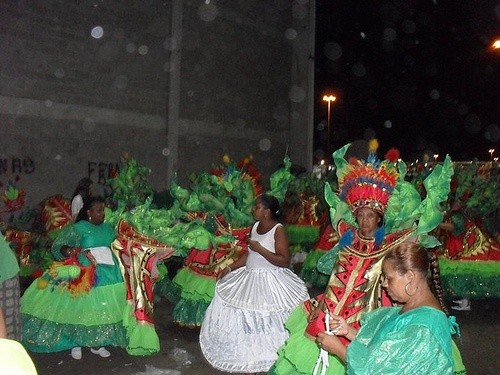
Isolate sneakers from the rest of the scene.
[71,347,82,359]
[91,347,111,357]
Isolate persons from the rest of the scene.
[0,143,500,375]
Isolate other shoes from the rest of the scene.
[452,300,470,310]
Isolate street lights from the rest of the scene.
[433,149,499,162]
[323,94,337,153]
